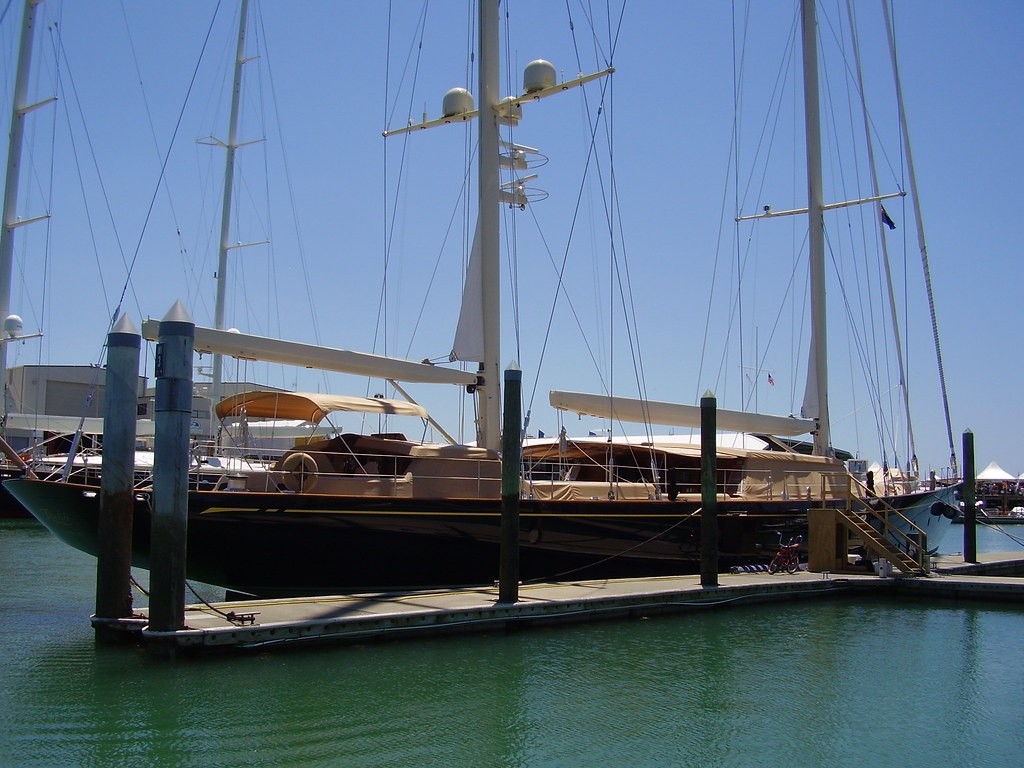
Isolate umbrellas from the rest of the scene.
[974,461,1024,481]
[880,205,897,230]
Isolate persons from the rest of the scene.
[976,482,1024,495]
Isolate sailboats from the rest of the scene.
[0,1,1024,598]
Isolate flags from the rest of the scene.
[767,374,775,386]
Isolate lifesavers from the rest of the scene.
[280,452,319,493]
